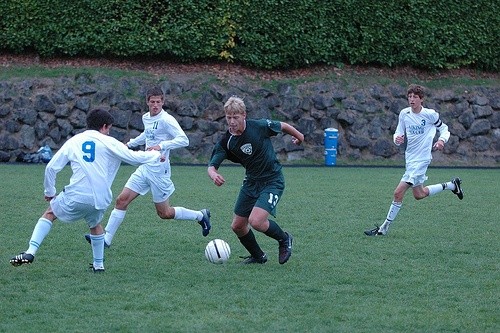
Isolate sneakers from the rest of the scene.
[85,234,110,250]
[89,263,105,274]
[364,226,386,237]
[278,231,293,265]
[450,176,465,200]
[237,251,268,265]
[198,209,211,237]
[9,252,35,267]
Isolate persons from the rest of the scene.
[9,108,167,274]
[364,83,464,237]
[85,88,211,250]
[207,97,304,266]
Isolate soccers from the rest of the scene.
[204,238,231,265]
